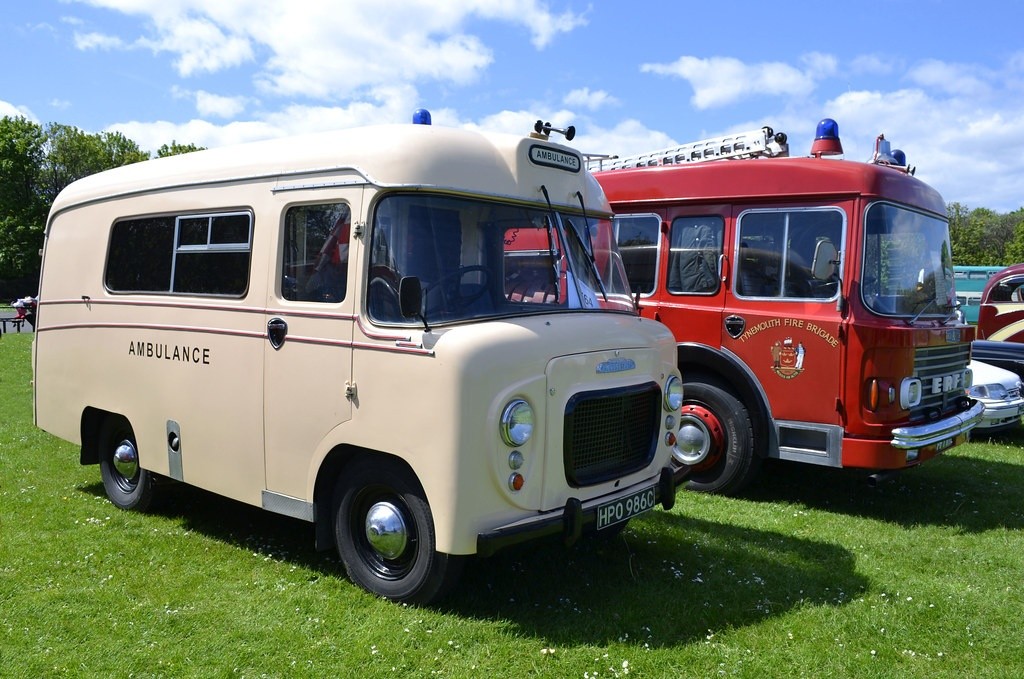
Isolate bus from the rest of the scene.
[31,105,684,602]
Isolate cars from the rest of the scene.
[916,265,1024,434]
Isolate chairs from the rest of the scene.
[450,284,496,318]
[370,266,403,323]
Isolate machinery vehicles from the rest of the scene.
[502,119,985,492]
